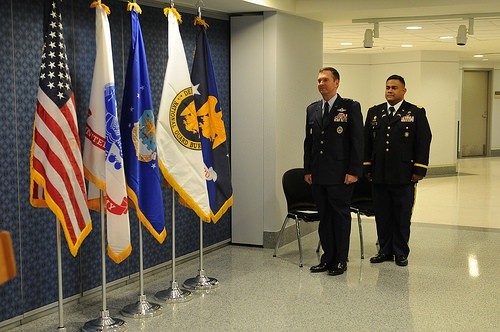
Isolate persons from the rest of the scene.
[304,67,365,276]
[363,74,431,266]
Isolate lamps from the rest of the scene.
[363,23,380,48]
[456,18,474,45]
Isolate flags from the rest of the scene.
[29,0,92,257]
[190,16,234,224]
[156,7,211,224]
[82,0,132,265]
[121,2,167,245]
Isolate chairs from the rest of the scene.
[348,163,379,260]
[273,168,349,267]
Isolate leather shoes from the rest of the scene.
[395,255,408,266]
[369,251,394,263]
[309,261,329,273]
[327,261,347,276]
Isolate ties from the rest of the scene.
[389,106,395,121]
[323,101,329,119]
